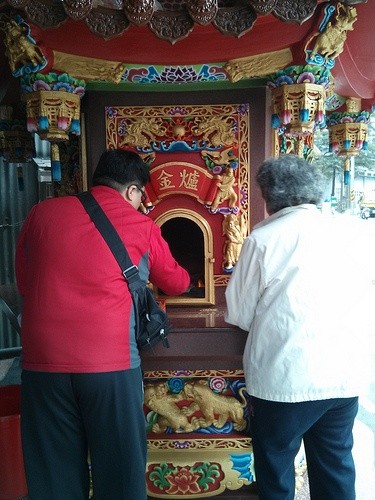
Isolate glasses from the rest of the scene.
[128,184,148,203]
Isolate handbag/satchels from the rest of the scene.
[74,190,172,352]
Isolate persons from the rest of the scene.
[16,145,189,500]
[225,153,374,500]
